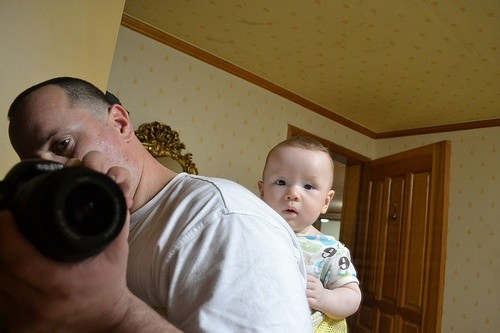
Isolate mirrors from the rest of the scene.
[134,121,199,176]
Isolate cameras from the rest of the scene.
[0,158,127,264]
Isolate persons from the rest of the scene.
[258,136,361,333]
[0,77,314,333]
